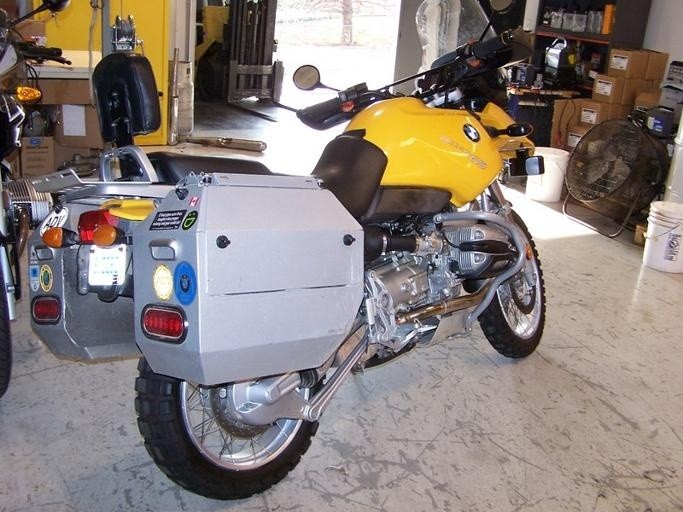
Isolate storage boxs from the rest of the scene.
[566,48,682,218]
[130,173,365,389]
[27,195,138,365]
[6,74,104,184]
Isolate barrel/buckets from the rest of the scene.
[642,200,682,276]
[544,42,573,70]
[526,147,569,202]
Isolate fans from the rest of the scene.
[557,117,671,240]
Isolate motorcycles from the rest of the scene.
[28,1,548,503]
[0,1,75,401]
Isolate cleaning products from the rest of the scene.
[601,2,616,35]
[549,8,602,34]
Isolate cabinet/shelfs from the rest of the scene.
[529,0,652,96]
